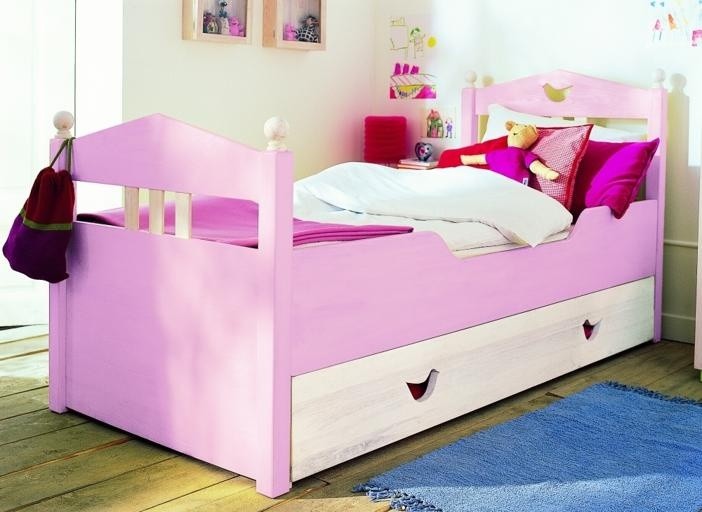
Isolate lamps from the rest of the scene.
[363,114,410,165]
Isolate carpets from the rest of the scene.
[349,378,701,511]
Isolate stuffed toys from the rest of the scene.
[202,1,320,43]
[460,121,560,188]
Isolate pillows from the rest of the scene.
[436,103,662,220]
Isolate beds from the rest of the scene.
[45,66,672,499]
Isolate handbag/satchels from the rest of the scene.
[1,138,75,284]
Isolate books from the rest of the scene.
[399,157,440,167]
[396,164,433,170]
